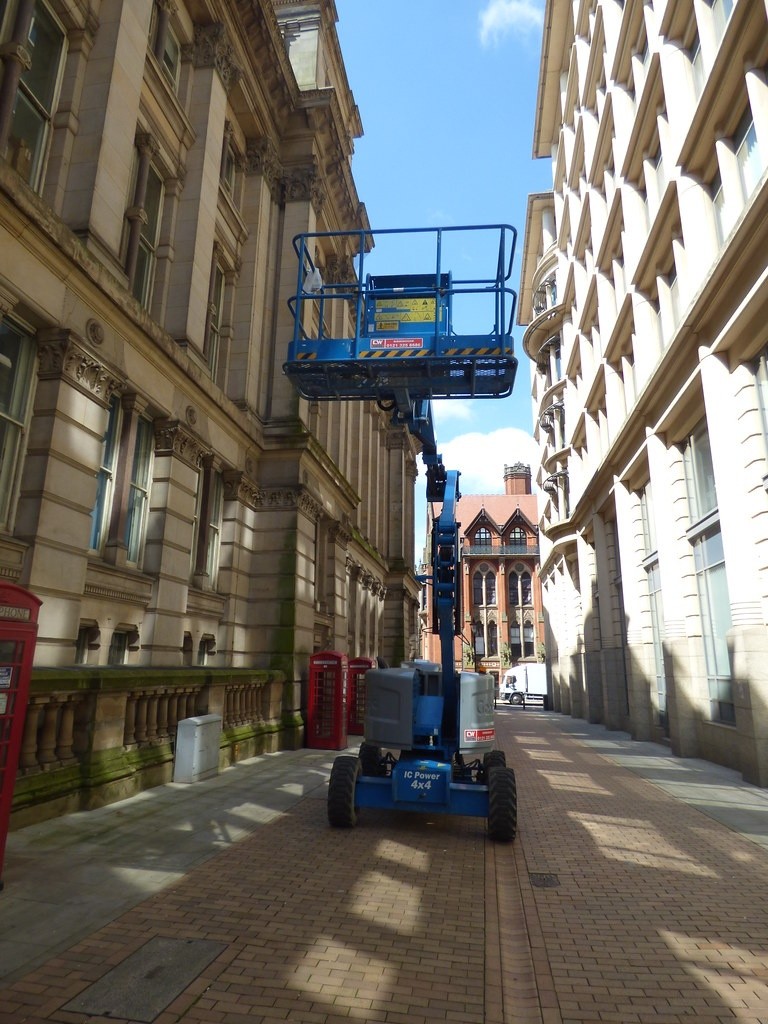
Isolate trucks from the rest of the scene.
[500,663,546,705]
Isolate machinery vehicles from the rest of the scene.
[281,221,518,845]
